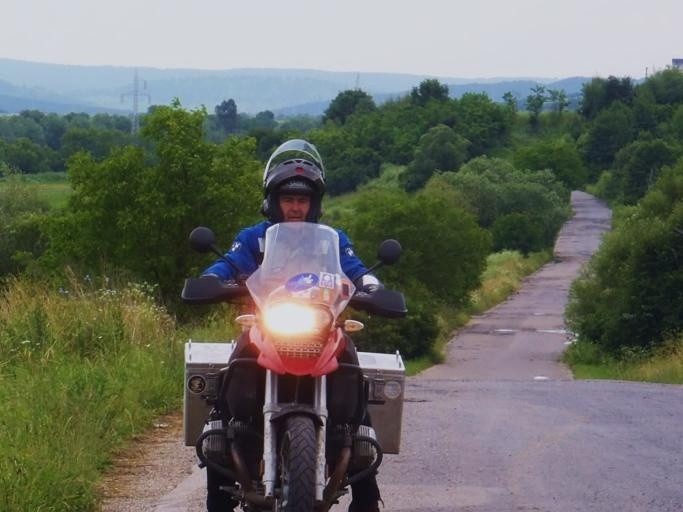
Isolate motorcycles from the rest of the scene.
[180,224,407,511]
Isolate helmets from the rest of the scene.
[261,139,325,222]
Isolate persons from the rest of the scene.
[200,138,384,512]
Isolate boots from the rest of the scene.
[348,468,384,510]
[242,481,258,512]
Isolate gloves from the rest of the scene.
[354,276,385,298]
